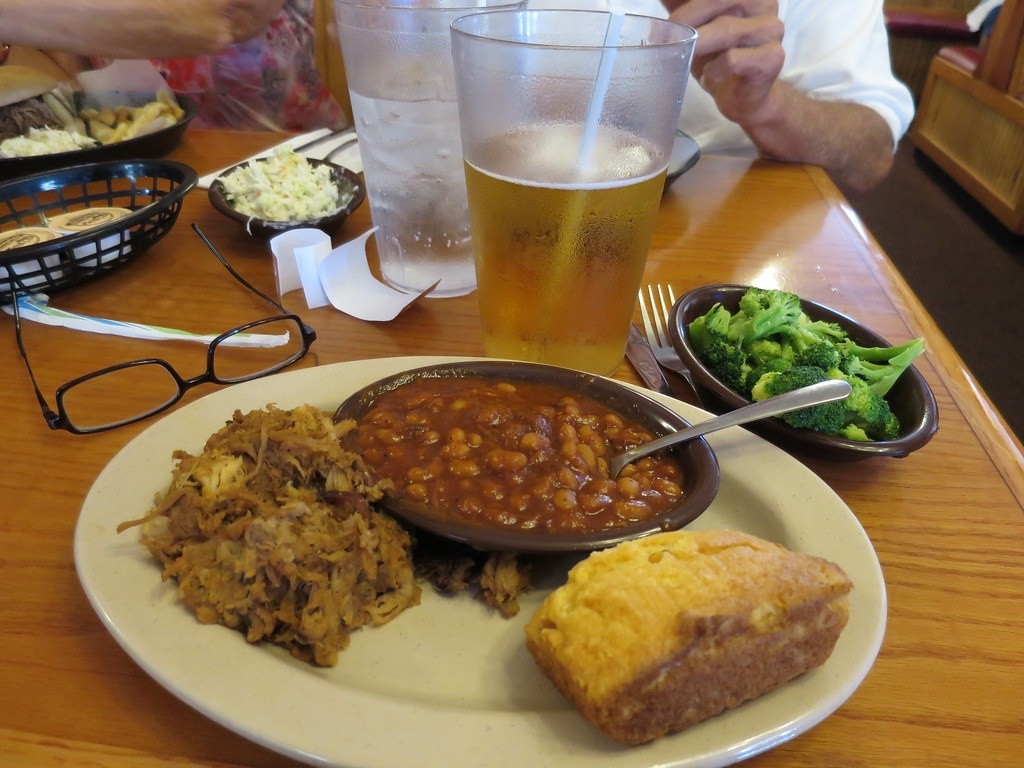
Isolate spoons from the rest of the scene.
[609,380,852,483]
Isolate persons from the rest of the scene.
[0,0,348,132]
[438,0,916,199]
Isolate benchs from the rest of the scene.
[883,0,1024,239]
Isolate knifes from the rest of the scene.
[625,322,675,399]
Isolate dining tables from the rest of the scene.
[0,128,1024,768]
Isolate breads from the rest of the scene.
[524,529,855,746]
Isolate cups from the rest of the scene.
[450,8,699,377]
[333,0,529,299]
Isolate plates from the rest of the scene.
[72,356,888,768]
[0,91,200,180]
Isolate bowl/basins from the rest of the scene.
[208,157,367,238]
[668,284,939,462]
[331,361,721,552]
[625,120,701,198]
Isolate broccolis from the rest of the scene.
[687,288,926,442]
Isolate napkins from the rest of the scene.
[196,129,363,191]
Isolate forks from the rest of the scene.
[638,283,713,415]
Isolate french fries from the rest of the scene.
[77,85,184,145]
